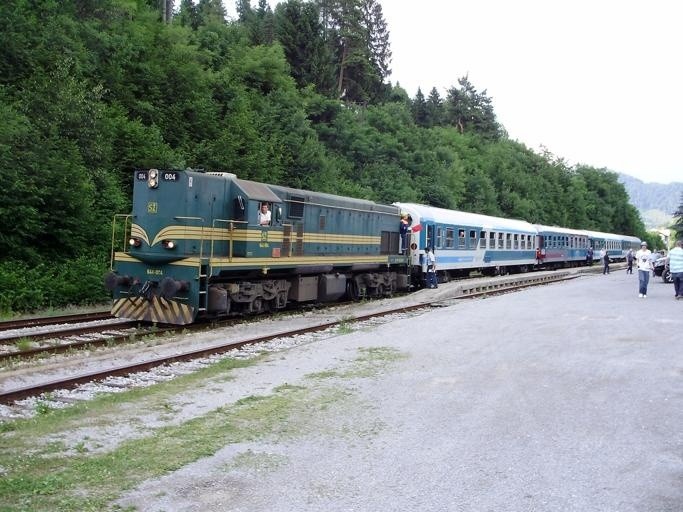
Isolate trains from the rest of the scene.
[104,166,642,324]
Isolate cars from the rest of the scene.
[656,258,674,283]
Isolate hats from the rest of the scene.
[640,241,648,246]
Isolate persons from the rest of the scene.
[424,247,438,289]
[400,214,412,255]
[627,241,683,300]
[536,247,544,266]
[586,246,594,266]
[599,247,607,265]
[603,251,611,274]
[259,203,271,226]
[410,242,423,291]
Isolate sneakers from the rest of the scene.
[639,293,646,298]
